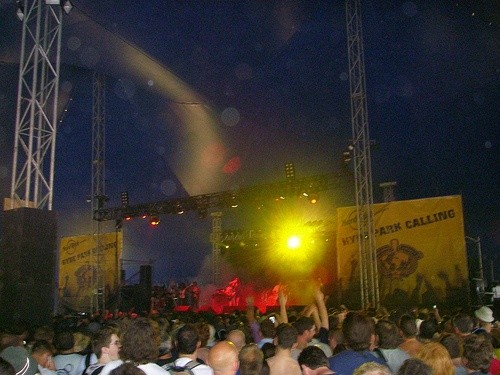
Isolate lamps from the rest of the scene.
[60,0,73,16]
[16,5,24,21]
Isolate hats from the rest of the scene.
[475,306,495,323]
[72,331,90,352]
[0,345,38,375]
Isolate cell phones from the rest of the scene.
[269,316,275,323]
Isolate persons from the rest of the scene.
[0,276,500,375]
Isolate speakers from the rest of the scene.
[140,265,152,288]
[0,207,56,336]
